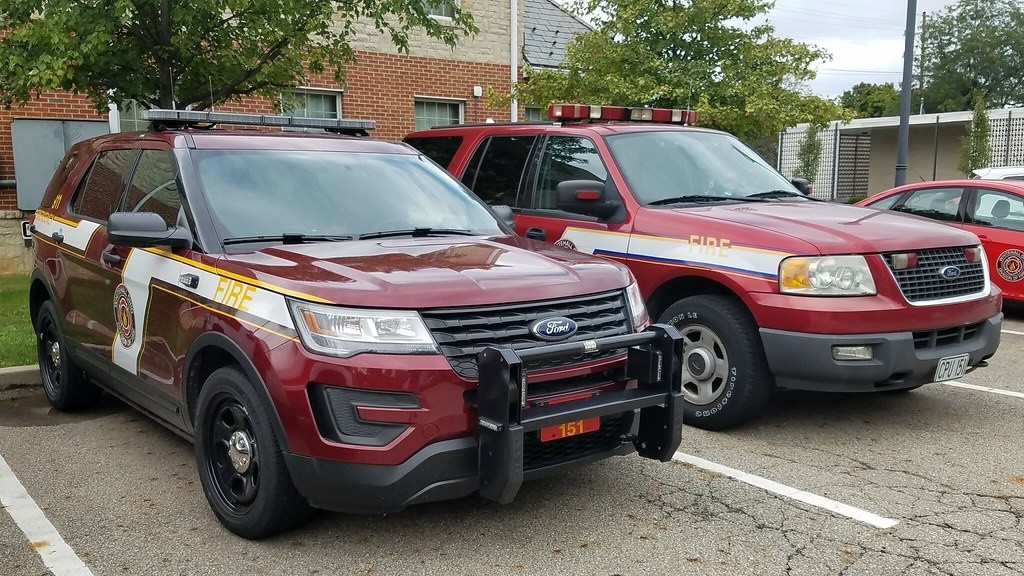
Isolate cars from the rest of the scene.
[961,166,1024,218]
[852,178,1024,305]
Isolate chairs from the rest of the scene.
[930,199,948,211]
[990,199,1010,219]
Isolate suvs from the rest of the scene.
[29,102,688,542]
[393,103,1005,433]
